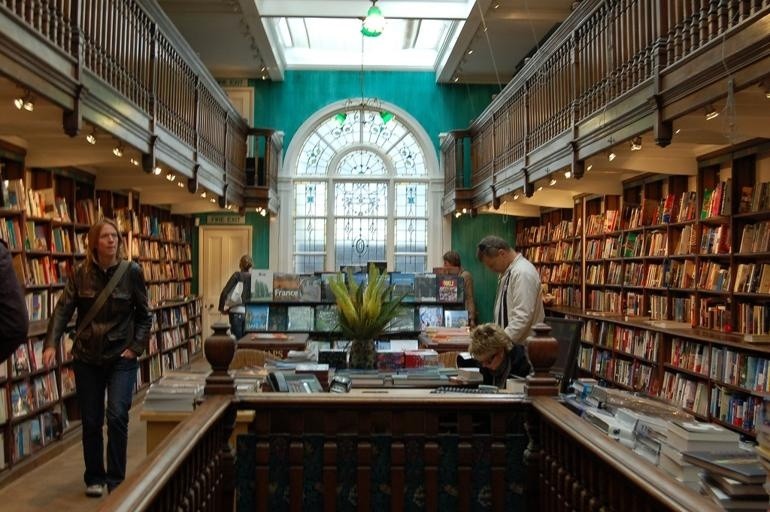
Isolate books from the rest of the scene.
[228,269,483,392]
[506,180,770,511]
[1,177,206,470]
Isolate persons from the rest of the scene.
[218,255,253,340]
[470,323,535,389]
[42,216,153,496]
[443,251,477,329]
[477,235,546,346]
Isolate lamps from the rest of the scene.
[360,0,385,38]
[84,123,97,146]
[671,127,680,136]
[335,36,396,129]
[703,102,720,121]
[113,137,124,159]
[758,79,770,100]
[14,85,34,112]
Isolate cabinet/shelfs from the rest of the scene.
[2,153,203,493]
[242,269,467,354]
[516,137,770,445]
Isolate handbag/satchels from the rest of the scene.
[227,282,243,307]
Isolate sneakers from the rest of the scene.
[84,484,104,496]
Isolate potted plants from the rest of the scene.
[325,257,412,369]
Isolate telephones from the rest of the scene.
[267,370,324,392]
